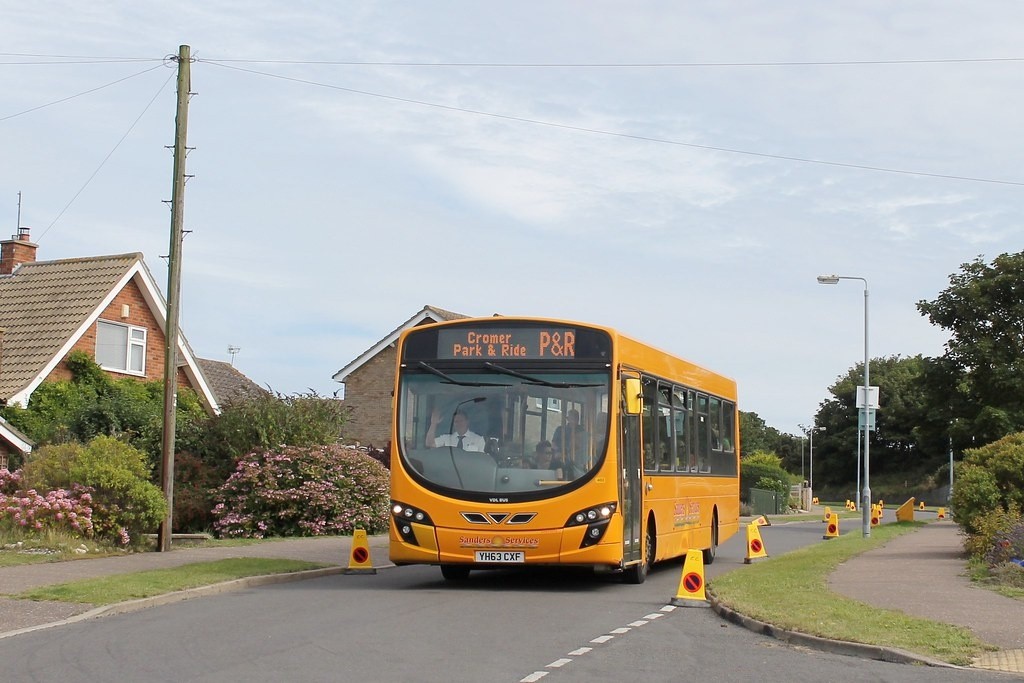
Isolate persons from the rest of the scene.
[643,439,695,471]
[722,427,731,451]
[426,409,485,453]
[552,409,585,448]
[531,440,563,470]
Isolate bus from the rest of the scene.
[387,314,742,584]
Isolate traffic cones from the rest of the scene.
[812,497,815,505]
[751,513,771,527]
[821,507,832,523]
[871,500,884,529]
[919,502,925,512]
[823,514,839,539]
[815,497,819,505]
[850,501,856,512]
[343,530,378,575]
[669,548,712,609]
[937,508,945,519]
[845,500,851,508]
[743,525,770,564]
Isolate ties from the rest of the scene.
[457,436,466,449]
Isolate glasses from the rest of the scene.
[677,445,686,448]
[542,451,552,455]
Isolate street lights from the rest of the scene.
[816,274,871,539]
[948,417,958,518]
[810,427,826,489]
